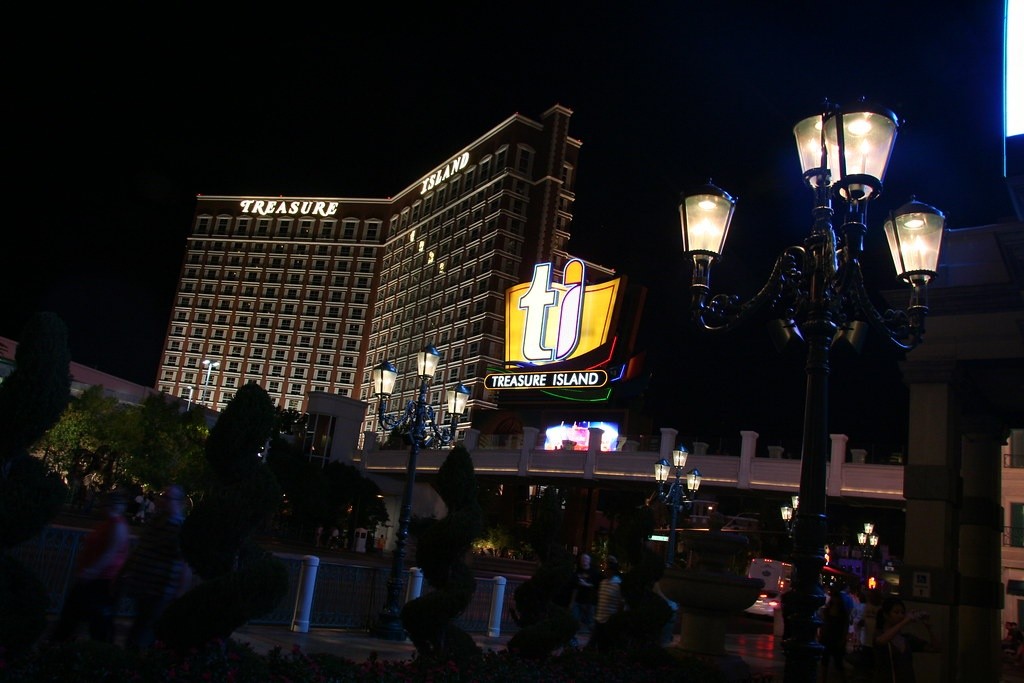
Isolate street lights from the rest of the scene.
[676,75,947,683]
[200,358,222,403]
[187,381,197,412]
[654,444,702,560]
[782,493,810,563]
[855,520,881,580]
[371,344,470,644]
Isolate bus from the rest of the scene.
[742,558,799,620]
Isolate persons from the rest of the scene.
[872,598,939,683]
[1003,622,1024,672]
[377,535,386,558]
[568,554,622,649]
[49,492,132,643]
[818,582,883,683]
[132,497,146,523]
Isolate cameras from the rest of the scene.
[914,610,927,620]
